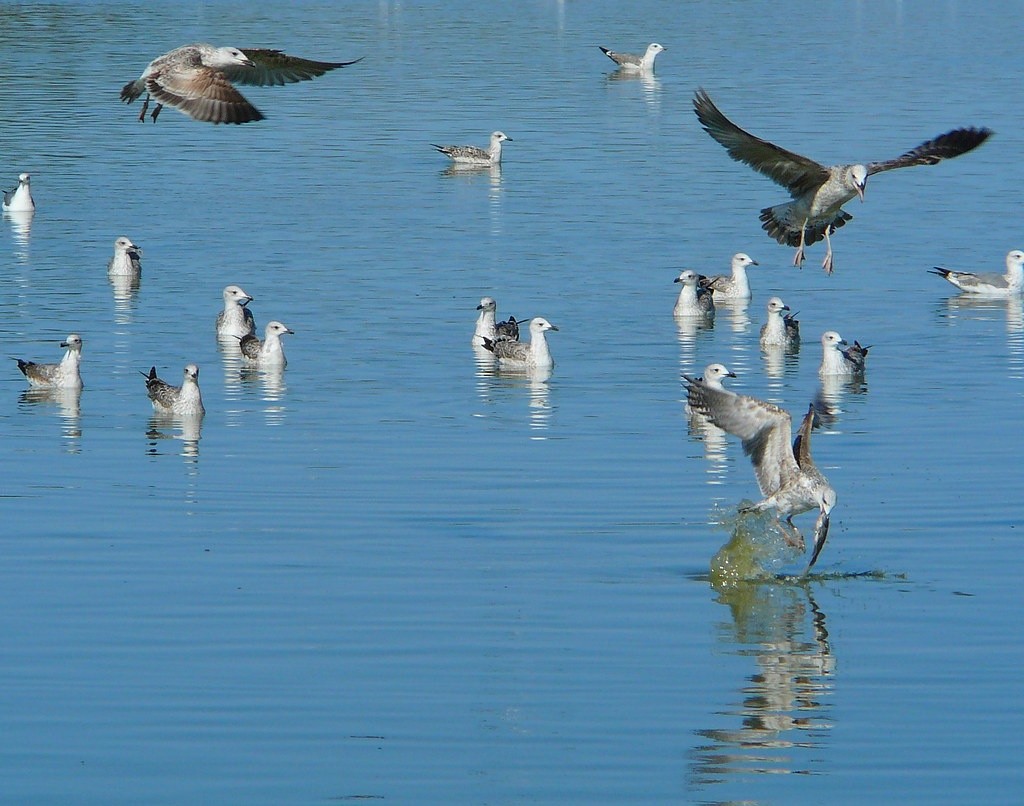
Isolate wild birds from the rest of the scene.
[472,296,561,369]
[433,132,513,166]
[8,335,85,390]
[214,284,294,373]
[121,41,367,127]
[600,42,670,69]
[818,329,872,378]
[108,237,145,277]
[681,362,838,576]
[761,296,804,348]
[4,172,34,212]
[691,84,997,276]
[673,254,760,318]
[925,250,1024,295]
[141,363,206,415]
